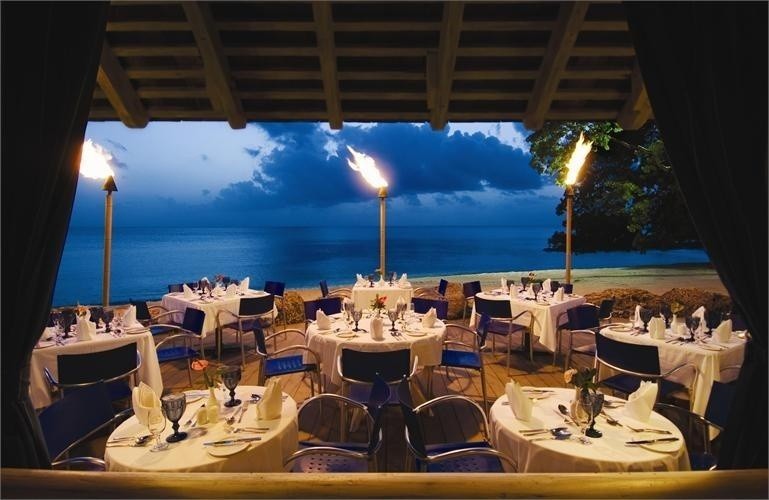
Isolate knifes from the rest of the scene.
[624,437,681,448]
[202,437,262,447]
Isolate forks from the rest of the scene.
[623,424,674,435]
[223,393,289,434]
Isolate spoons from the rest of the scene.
[558,403,581,426]
[105,435,153,445]
[518,427,572,443]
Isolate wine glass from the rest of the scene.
[146,409,170,451]
[637,304,700,344]
[366,272,394,288]
[160,392,189,443]
[549,280,557,296]
[220,366,242,408]
[343,302,363,332]
[388,303,407,332]
[507,277,542,302]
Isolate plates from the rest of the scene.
[208,435,251,457]
[523,386,551,399]
[608,326,632,332]
[408,331,426,336]
[633,432,680,453]
[185,389,204,403]
[336,332,356,338]
[700,343,730,352]
